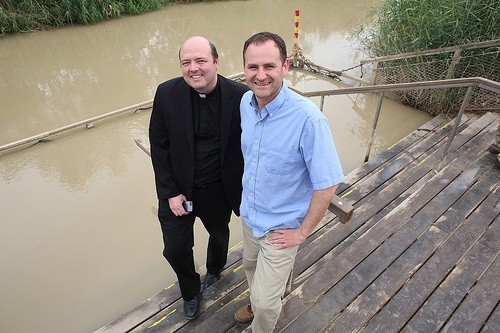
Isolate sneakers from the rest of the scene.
[234,304,254,323]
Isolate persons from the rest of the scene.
[233,32,346,333]
[148,35,250,321]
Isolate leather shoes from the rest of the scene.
[183,292,200,320]
[203,276,218,288]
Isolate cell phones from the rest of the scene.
[182,201,194,212]
